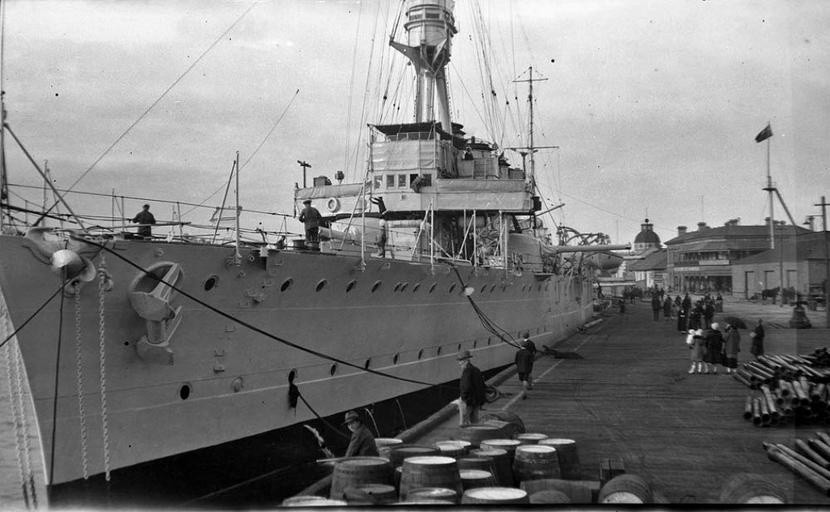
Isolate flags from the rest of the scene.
[754,127,773,142]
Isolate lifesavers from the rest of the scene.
[326,197,337,212]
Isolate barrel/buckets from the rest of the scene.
[329,414,671,505]
[718,471,789,503]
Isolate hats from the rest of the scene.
[524,332,529,338]
[520,340,528,347]
[303,199,311,204]
[344,410,359,424]
[711,322,720,331]
[455,351,473,361]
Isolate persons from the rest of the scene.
[344,412,379,459]
[515,340,532,399]
[596,283,767,375]
[299,200,322,242]
[369,197,389,220]
[455,350,487,425]
[374,218,386,257]
[276,236,286,250]
[465,147,474,160]
[133,205,156,237]
[520,332,537,355]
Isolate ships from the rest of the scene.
[0,1,633,511]
